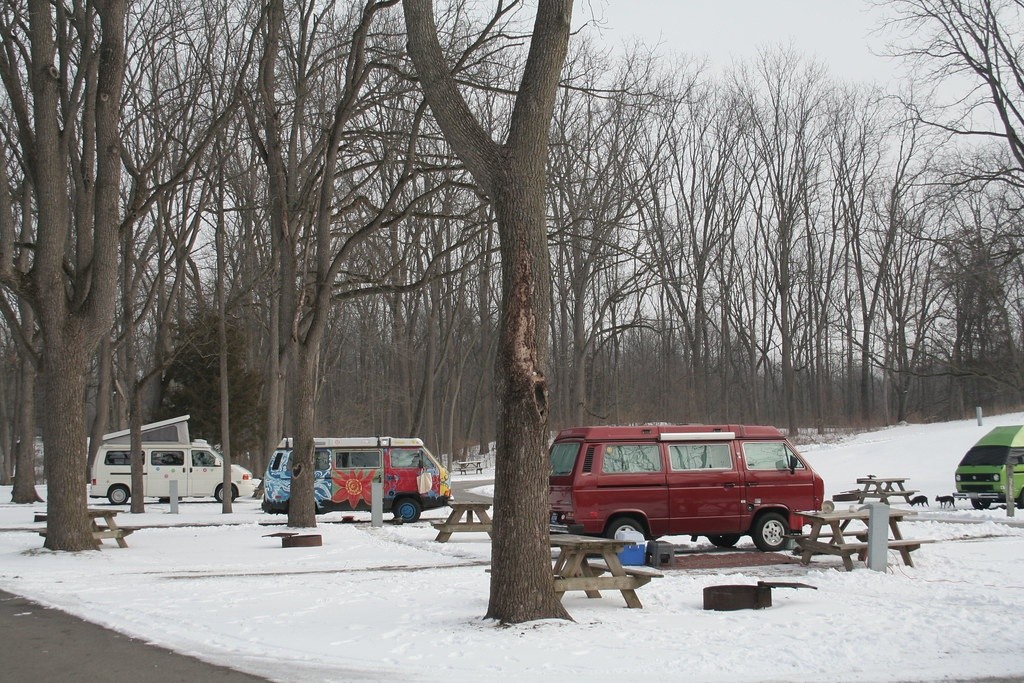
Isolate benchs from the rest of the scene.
[879,490,920,507]
[587,562,664,608]
[830,539,936,572]
[429,519,452,542]
[848,489,887,503]
[39,530,132,548]
[780,530,868,567]
[92,524,141,545]
[458,461,483,475]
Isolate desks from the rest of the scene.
[793,508,915,572]
[433,500,493,543]
[35,508,130,548]
[853,477,915,506]
[549,534,643,609]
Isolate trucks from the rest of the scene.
[953,426,1024,509]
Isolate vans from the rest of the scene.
[261,436,451,522]
[90,443,253,505]
[545,424,823,552]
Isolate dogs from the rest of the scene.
[909,496,929,507]
[935,495,955,509]
